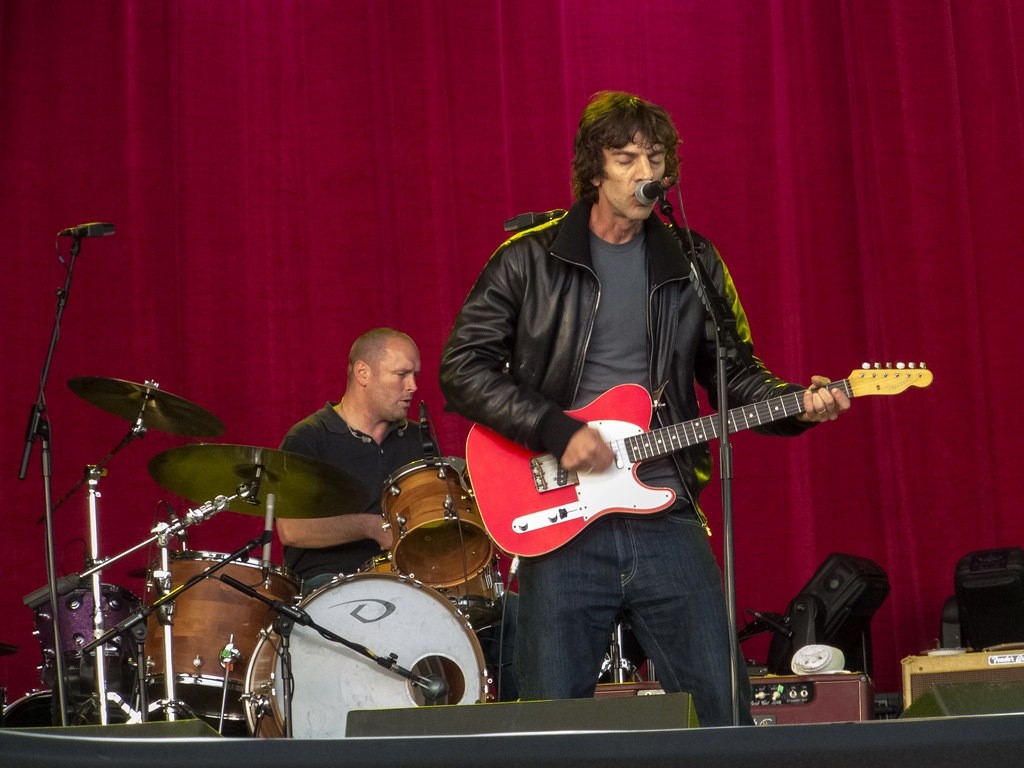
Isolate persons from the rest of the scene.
[437,88,850,729]
[274,329,518,701]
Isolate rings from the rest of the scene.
[819,408,826,413]
[588,467,593,473]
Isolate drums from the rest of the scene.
[360,551,502,627]
[242,570,490,738]
[381,455,494,588]
[144,549,304,721]
[2,689,141,728]
[32,579,148,699]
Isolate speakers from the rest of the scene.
[0,718,224,739]
[899,648,1024,719]
[344,692,701,731]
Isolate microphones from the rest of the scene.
[635,176,677,205]
[504,209,567,232]
[58,222,116,237]
[418,402,434,451]
[422,675,449,700]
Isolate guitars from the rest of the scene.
[464,357,935,563]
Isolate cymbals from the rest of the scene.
[65,373,228,438]
[146,442,373,520]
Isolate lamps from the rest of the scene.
[940,545,1024,647]
[738,553,890,675]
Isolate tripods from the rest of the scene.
[38,392,264,727]
